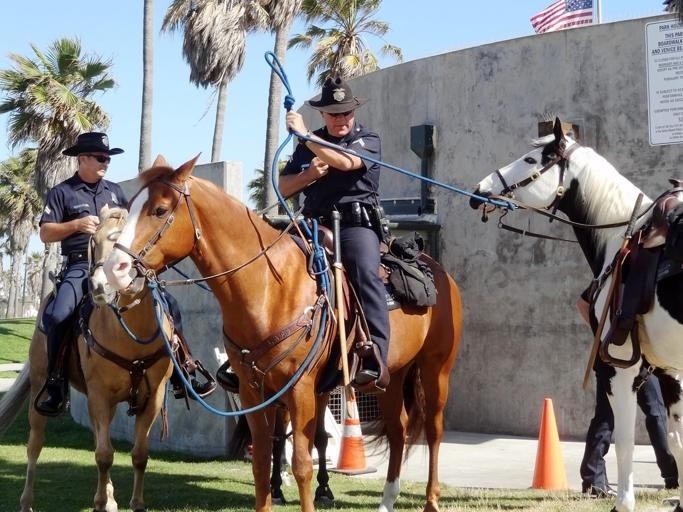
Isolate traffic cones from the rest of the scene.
[517,397,577,494]
[326,386,377,476]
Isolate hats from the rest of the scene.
[62,132,124,156]
[305,77,369,114]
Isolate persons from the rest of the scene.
[217,77,388,394]
[35,133,215,412]
[577,276,678,498]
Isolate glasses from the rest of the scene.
[89,155,109,162]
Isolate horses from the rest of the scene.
[467,115,683,512]
[101,152,464,512]
[18,203,179,512]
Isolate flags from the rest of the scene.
[529,1,592,35]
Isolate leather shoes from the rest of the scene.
[216,372,238,388]
[583,486,616,496]
[355,369,386,394]
[173,380,215,399]
[38,394,63,413]
